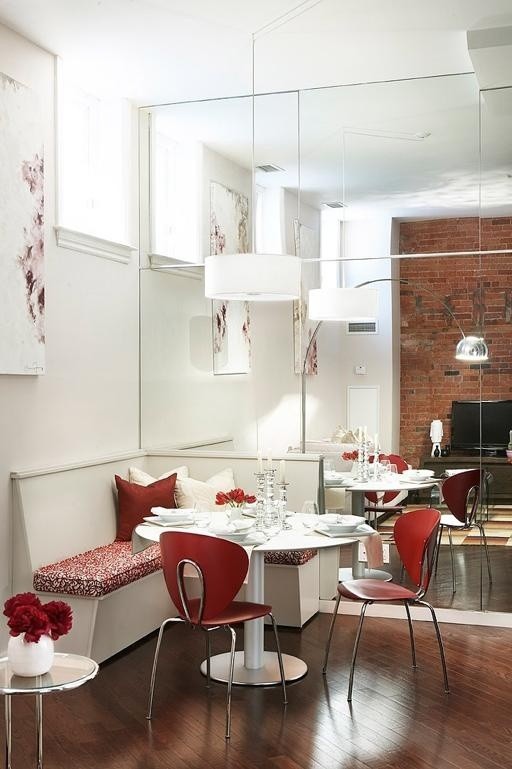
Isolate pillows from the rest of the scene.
[127,466,188,485]
[114,474,176,542]
[170,468,234,508]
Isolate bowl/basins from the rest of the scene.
[215,532,248,541]
[150,506,196,522]
[402,468,436,481]
[324,479,344,485]
[328,523,359,533]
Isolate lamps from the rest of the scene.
[204,39,306,299]
[303,128,374,321]
[300,278,487,455]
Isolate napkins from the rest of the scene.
[225,520,256,532]
[149,506,185,515]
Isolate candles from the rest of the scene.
[255,447,287,484]
[358,424,380,455]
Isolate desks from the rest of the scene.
[326,476,438,583]
[137,513,361,686]
[0,653,98,769]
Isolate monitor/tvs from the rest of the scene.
[450,399,511,457]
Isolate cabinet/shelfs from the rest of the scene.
[418,454,512,506]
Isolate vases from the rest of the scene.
[230,508,243,522]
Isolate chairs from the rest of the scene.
[145,529,286,739]
[361,454,410,524]
[320,509,449,703]
[432,469,493,588]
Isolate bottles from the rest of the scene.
[434,445,450,457]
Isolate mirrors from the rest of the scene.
[139,72,512,613]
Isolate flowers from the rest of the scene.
[215,489,253,507]
[3,589,73,642]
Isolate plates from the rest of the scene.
[403,477,445,486]
[314,524,375,538]
[143,516,195,527]
[323,484,354,489]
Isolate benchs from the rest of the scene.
[8,449,342,664]
